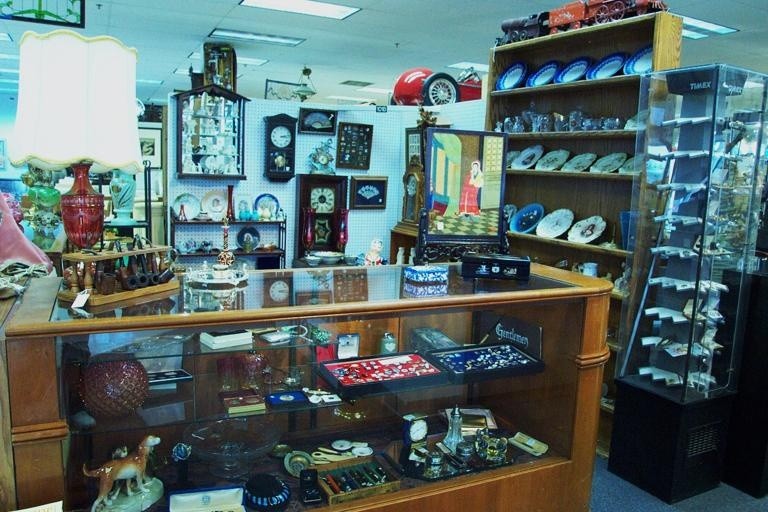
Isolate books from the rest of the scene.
[200,329,253,350]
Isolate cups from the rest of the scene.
[505,112,620,133]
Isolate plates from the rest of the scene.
[568,215,607,244]
[505,146,646,173]
[535,208,574,239]
[509,203,545,234]
[624,109,651,129]
[496,47,653,90]
[172,192,280,249]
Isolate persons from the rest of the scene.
[459,160,484,214]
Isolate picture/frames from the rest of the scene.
[423,128,509,245]
[298,107,338,135]
[335,121,373,169]
[349,176,388,210]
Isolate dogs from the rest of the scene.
[82,434,162,512]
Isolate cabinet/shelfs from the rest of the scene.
[170,207,287,268]
[4,260,615,512]
[485,11,684,400]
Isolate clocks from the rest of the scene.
[294,173,349,265]
[399,156,426,231]
[262,271,294,308]
[264,111,297,183]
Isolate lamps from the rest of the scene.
[7,29,145,289]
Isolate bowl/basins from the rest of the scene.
[304,252,359,268]
